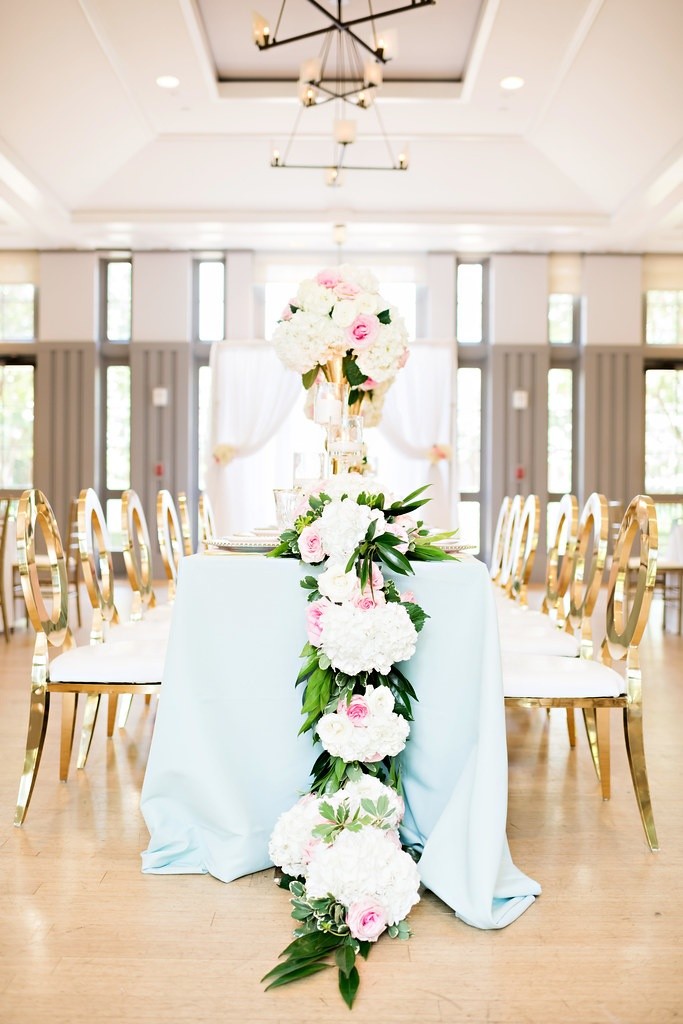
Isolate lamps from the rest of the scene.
[250,0,437,189]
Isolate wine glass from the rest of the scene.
[312,380,364,483]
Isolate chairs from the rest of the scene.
[10,487,221,829]
[486,491,661,857]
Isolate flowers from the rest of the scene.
[260,263,461,1014]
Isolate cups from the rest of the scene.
[293,452,325,491]
[275,488,315,537]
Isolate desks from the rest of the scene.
[136,547,542,930]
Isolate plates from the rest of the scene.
[432,542,477,552]
[202,538,280,551]
